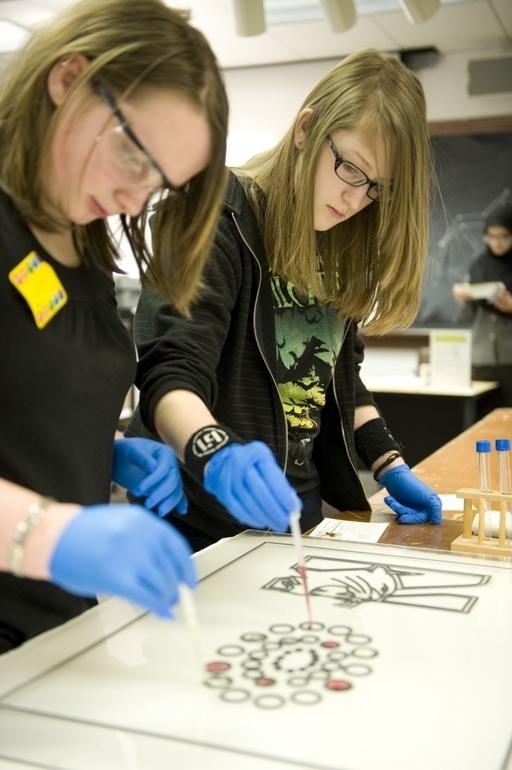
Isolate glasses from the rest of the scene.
[325,132,394,206]
[98,85,191,208]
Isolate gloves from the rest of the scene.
[50,503,196,619]
[111,437,188,517]
[202,440,303,532]
[378,463,442,525]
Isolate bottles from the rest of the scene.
[478,440,512,546]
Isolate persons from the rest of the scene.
[1,0,231,663]
[129,48,447,545]
[452,211,512,418]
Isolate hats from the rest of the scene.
[485,204,511,234]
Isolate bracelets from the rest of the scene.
[182,424,247,487]
[10,492,56,577]
[368,452,406,482]
[353,416,402,469]
